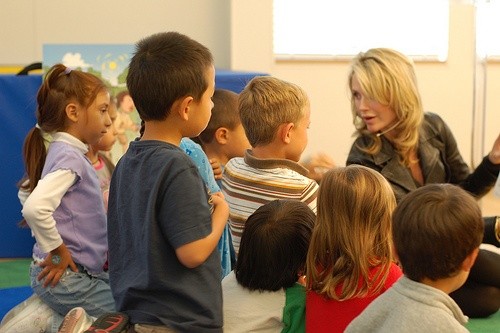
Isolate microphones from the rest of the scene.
[376,121,401,137]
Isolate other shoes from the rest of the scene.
[135,323,176,333]
[84,311,130,333]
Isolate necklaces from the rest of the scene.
[92,160,100,165]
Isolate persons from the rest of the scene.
[305,164,404,333]
[219,200,318,333]
[17,64,118,333]
[343,183,484,333]
[348,48,500,317]
[83,87,255,279]
[83,32,231,333]
[221,77,321,263]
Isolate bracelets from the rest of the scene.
[494,217,500,242]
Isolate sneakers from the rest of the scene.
[0,293,54,333]
[57,307,93,333]
